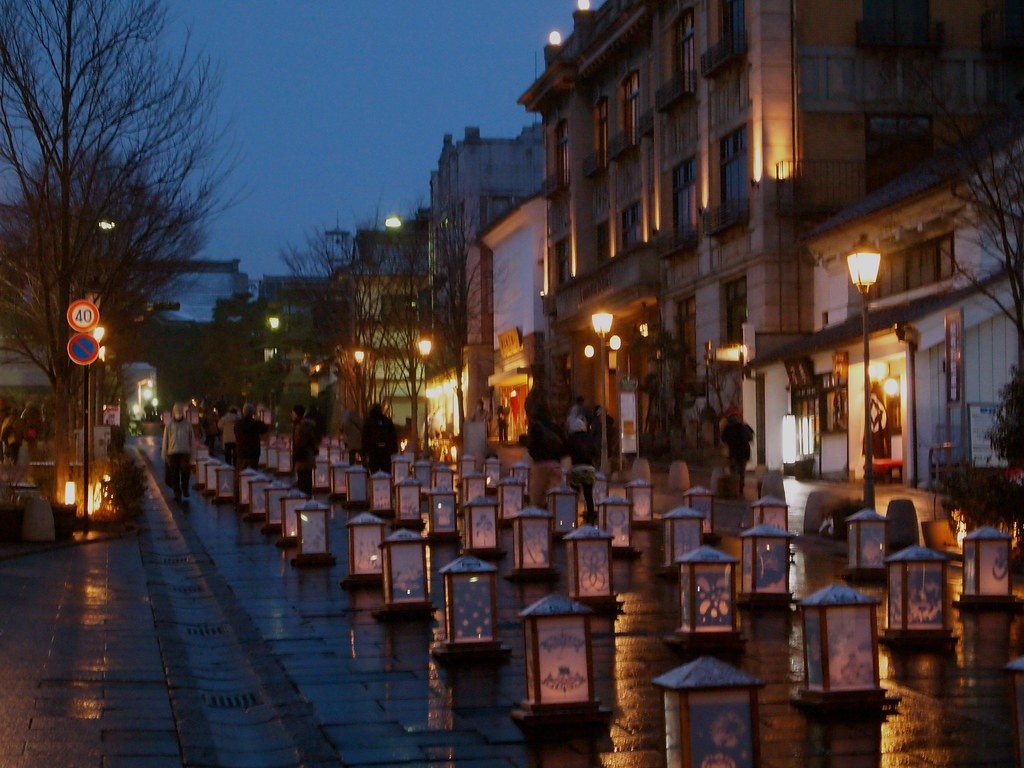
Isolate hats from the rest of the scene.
[10,407,20,414]
[293,405,306,418]
[724,408,739,417]
[25,401,35,408]
[173,402,184,414]
[243,403,255,414]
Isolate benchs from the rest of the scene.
[4,483,55,543]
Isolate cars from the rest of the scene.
[275,402,326,438]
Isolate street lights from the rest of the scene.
[845,234,882,509]
[270,316,279,358]
[591,310,613,471]
[418,340,431,460]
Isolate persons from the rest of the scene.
[568,406,615,465]
[162,403,195,502]
[234,404,267,470]
[0,402,41,463]
[217,409,239,465]
[290,406,318,495]
[497,405,504,440]
[203,406,219,457]
[721,414,753,493]
[361,403,398,474]
[144,403,155,421]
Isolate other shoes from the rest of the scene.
[172,484,180,500]
[181,488,190,502]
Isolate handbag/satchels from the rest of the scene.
[164,463,172,488]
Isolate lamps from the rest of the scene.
[191,424,1024,768]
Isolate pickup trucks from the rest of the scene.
[139,415,165,434]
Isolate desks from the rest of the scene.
[24,462,86,482]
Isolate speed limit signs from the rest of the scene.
[67,300,100,333]
